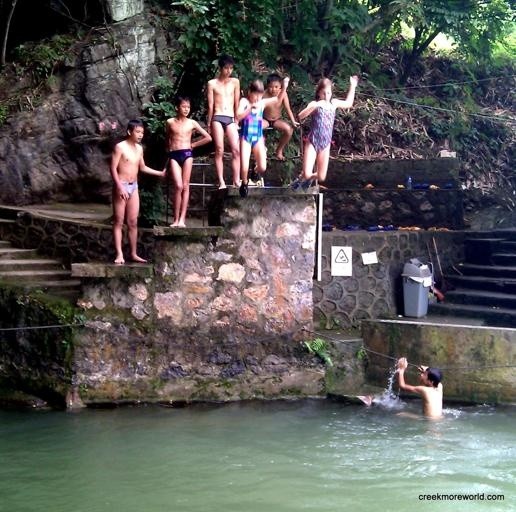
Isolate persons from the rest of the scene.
[291,75,359,193]
[263,74,300,161]
[166,97,212,227]
[236,77,290,198]
[397,358,444,422]
[207,56,241,190]
[110,121,167,265]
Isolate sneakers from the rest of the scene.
[251,162,258,183]
[302,180,313,193]
[239,181,247,198]
[291,174,304,193]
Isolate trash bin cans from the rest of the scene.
[401,258,433,318]
